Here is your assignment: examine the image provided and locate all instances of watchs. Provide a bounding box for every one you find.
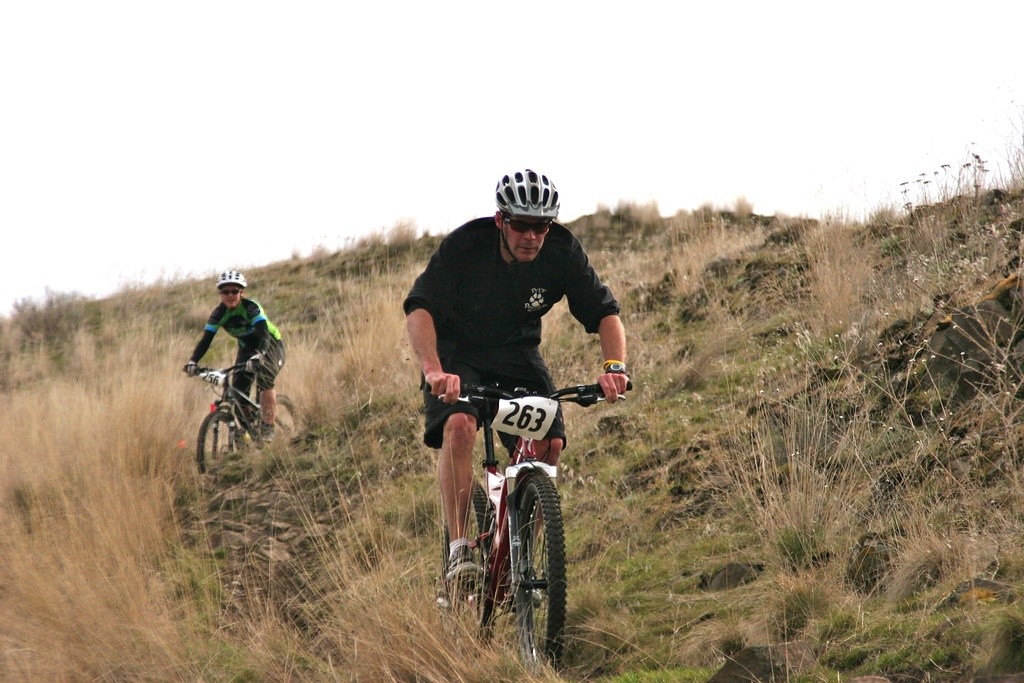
[605,364,626,374]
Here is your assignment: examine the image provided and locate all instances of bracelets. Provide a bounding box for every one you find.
[603,360,626,369]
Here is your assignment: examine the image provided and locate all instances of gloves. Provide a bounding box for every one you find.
[182,360,197,378]
[245,352,266,373]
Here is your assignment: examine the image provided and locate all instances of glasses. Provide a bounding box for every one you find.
[220,289,240,295]
[503,216,553,235]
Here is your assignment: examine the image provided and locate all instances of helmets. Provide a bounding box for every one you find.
[495,168,560,222]
[216,271,247,289]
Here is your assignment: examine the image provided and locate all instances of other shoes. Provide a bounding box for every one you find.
[259,422,275,443]
[223,445,240,457]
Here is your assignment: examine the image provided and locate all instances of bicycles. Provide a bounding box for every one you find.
[182,358,299,478]
[418,374,634,671]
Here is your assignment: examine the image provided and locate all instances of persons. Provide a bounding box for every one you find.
[402,168,632,589]
[183,270,285,436]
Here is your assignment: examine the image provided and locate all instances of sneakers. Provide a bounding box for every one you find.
[444,545,483,593]
[509,566,541,613]
